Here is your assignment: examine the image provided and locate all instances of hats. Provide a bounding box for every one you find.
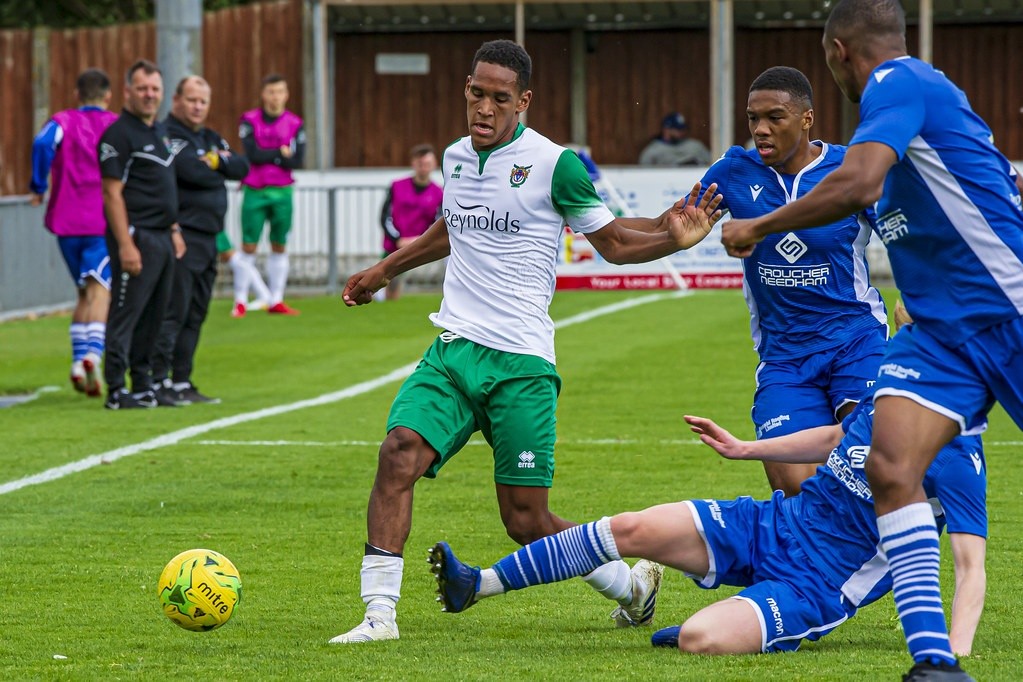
[663,113,687,129]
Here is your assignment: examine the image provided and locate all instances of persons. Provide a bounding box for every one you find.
[378,145,444,301]
[324,40,723,645]
[426,300,988,659]
[612,1,1022,681]
[29,58,306,409]
[640,112,711,170]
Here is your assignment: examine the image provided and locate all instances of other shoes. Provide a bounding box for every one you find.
[159,380,220,406]
[107,383,176,410]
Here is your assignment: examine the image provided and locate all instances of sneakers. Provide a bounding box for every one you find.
[232,302,245,317]
[610,559,663,627]
[268,302,300,315]
[652,624,682,648]
[329,608,399,644]
[902,659,976,682]
[247,300,268,310]
[427,542,482,614]
[70,353,102,397]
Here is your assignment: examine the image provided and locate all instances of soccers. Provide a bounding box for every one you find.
[157,548,242,633]
